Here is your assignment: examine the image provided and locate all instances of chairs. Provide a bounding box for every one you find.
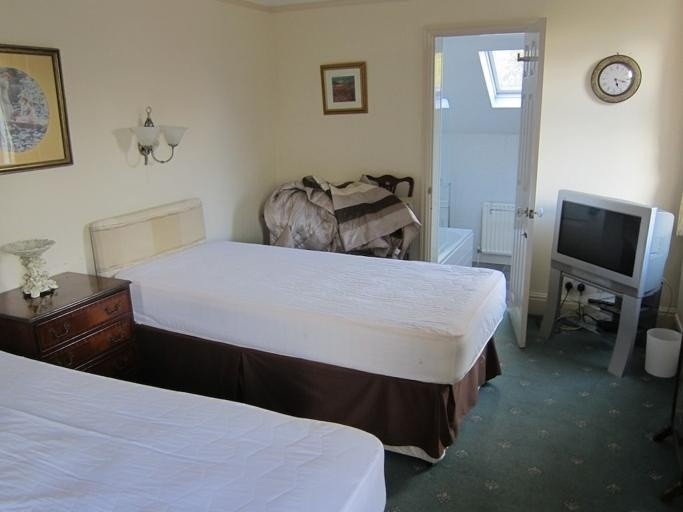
[357,174,415,260]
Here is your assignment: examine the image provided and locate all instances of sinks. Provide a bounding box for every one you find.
[438,224,472,262]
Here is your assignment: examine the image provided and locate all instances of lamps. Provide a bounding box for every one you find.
[128,105,189,167]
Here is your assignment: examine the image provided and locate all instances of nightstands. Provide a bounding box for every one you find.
[0,270,138,380]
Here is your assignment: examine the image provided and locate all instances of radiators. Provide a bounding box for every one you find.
[476,202,515,257]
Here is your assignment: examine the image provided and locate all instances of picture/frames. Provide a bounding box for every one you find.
[0,43,74,176]
[319,62,368,116]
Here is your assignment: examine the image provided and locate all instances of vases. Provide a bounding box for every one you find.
[0,237,57,298]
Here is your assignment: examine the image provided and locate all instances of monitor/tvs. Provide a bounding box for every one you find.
[550,188,674,300]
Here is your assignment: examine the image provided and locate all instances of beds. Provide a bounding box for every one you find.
[88,197,509,464]
[0,348,386,510]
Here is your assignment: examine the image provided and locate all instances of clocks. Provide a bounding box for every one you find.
[590,55,642,105]
